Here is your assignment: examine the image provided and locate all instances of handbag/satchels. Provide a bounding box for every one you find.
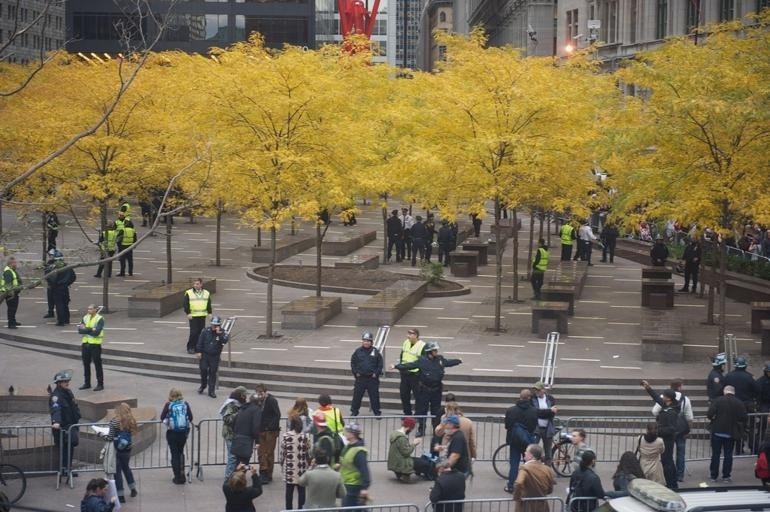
[113,431,131,451]
[511,422,532,449]
[660,413,689,441]
[230,434,254,460]
[732,420,744,439]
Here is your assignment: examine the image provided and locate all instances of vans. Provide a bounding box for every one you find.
[591,477,770,512]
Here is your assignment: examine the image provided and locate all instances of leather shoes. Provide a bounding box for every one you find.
[79,383,90,389]
[93,384,104,390]
[678,288,688,291]
[9,325,17,328]
[690,288,696,293]
[66,472,78,477]
[198,385,207,393]
[44,312,53,317]
[209,392,216,397]
[16,322,21,325]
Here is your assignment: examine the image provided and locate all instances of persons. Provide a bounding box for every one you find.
[529,238,551,302]
[47,252,77,326]
[43,248,62,318]
[382,207,459,267]
[45,371,82,478]
[160,388,194,484]
[350,328,462,436]
[77,477,116,512]
[46,211,61,253]
[221,384,477,512]
[93,203,137,278]
[97,402,137,502]
[77,303,104,392]
[184,279,229,398]
[505,353,770,511]
[0,256,22,329]
[468,209,483,237]
[559,216,770,294]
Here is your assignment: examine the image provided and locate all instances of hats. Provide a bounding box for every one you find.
[234,386,251,394]
[313,413,329,427]
[345,422,362,433]
[407,328,419,334]
[442,415,459,423]
[531,382,545,389]
[401,416,416,428]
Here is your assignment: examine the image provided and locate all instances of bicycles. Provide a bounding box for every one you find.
[491,424,589,480]
[0,461,26,512]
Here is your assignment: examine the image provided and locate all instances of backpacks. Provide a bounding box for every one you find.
[163,400,190,430]
[320,433,345,455]
[566,471,592,509]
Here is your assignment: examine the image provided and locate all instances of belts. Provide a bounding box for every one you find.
[419,385,442,392]
[538,426,546,429]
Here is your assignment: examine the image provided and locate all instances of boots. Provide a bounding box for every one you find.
[257,473,269,484]
[267,471,272,482]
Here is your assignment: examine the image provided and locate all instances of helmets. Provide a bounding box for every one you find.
[733,356,748,367]
[361,332,373,340]
[687,235,697,241]
[209,315,222,324]
[763,360,770,371]
[424,341,439,350]
[655,233,663,239]
[53,370,72,382]
[48,248,57,255]
[712,353,727,366]
[54,253,64,259]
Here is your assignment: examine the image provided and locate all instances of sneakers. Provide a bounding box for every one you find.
[118,495,126,503]
[130,489,137,497]
[710,477,717,482]
[722,477,731,482]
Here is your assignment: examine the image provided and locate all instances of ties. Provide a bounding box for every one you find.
[403,215,405,228]
